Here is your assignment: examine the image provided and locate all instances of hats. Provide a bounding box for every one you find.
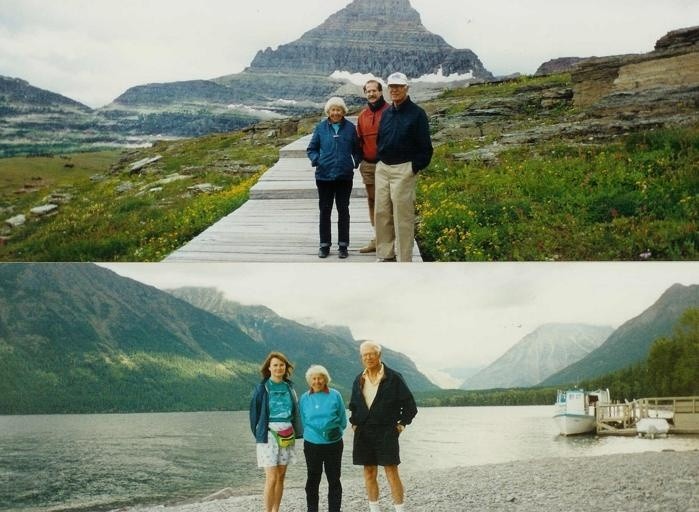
[387,72,408,85]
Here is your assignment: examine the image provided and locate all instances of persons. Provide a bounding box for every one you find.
[356,79,390,253]
[374,72,433,262]
[249,352,302,511]
[308,97,362,258]
[350,340,418,511]
[298,363,346,512]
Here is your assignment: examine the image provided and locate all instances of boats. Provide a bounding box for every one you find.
[551,389,612,436]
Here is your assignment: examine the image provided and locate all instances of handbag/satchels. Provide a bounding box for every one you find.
[268,426,297,448]
[306,424,341,441]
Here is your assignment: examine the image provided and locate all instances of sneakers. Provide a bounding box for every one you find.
[318,246,331,258]
[338,246,348,258]
[359,238,376,253]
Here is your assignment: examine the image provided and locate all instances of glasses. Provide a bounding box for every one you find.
[362,352,377,359]
